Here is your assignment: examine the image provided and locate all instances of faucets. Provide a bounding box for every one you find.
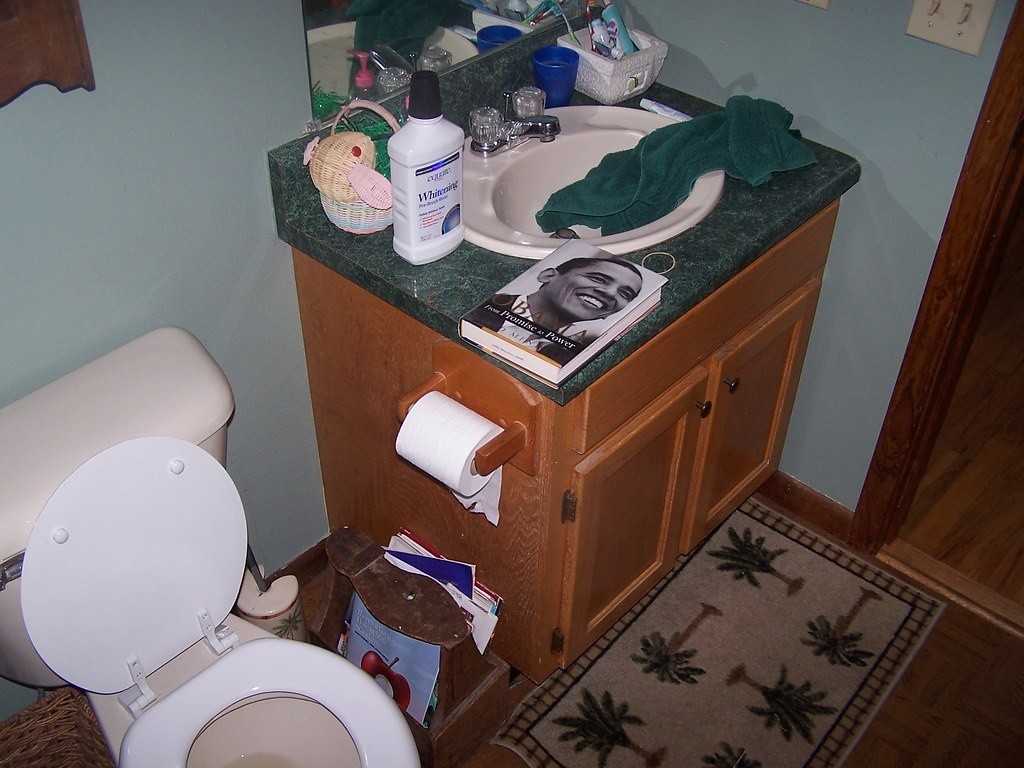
[366,42,454,96]
[465,82,563,159]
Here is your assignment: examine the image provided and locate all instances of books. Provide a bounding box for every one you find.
[457,238,670,389]
[338,527,503,727]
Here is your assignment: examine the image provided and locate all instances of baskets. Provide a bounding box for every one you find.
[320,100,401,234]
[308,132,376,203]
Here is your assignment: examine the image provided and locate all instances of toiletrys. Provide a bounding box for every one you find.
[340,48,377,103]
[383,68,470,268]
[638,97,695,122]
[446,23,478,42]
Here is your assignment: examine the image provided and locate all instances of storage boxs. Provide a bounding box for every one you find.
[309,523,510,768]
[556,25,667,105]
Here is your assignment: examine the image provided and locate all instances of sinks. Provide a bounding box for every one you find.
[306,18,480,99]
[460,101,729,263]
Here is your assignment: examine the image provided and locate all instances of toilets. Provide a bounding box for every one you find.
[0,322,427,768]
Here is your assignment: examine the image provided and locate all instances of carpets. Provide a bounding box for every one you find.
[484,493,948,768]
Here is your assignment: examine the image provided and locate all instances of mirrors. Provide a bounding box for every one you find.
[300,0,587,129]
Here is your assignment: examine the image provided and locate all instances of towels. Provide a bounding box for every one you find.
[532,94,821,239]
[0,0,98,110]
[343,0,463,96]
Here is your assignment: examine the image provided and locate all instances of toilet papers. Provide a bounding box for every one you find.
[392,389,506,528]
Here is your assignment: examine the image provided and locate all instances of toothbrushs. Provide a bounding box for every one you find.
[467,0,566,25]
[547,0,637,59]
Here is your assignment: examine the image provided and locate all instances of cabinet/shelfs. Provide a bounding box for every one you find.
[291,194,842,686]
